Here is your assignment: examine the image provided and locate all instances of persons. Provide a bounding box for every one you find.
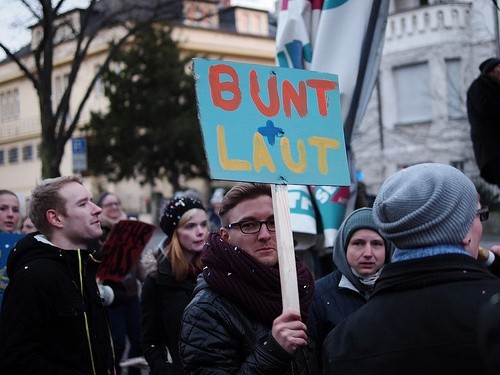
[321,163,500,375]
[0,175,117,375]
[467,58,500,188]
[20,216,38,234]
[0,190,28,307]
[311,207,394,338]
[178,182,319,375]
[98,192,141,375]
[139,195,211,375]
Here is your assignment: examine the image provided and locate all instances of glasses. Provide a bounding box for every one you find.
[476,206,490,221]
[226,217,276,234]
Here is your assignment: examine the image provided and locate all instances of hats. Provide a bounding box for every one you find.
[159,194,205,236]
[373,161,478,246]
[332,207,390,247]
